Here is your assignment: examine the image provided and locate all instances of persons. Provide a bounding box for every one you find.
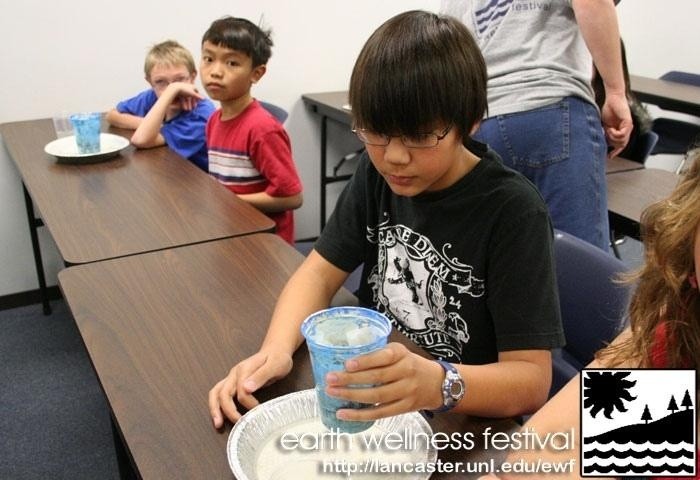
[205,10,567,429]
[591,37,653,239]
[106,40,216,173]
[448,0,634,253]
[473,143,700,480]
[198,16,303,247]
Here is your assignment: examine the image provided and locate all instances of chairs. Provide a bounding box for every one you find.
[641,129,660,165]
[257,100,288,126]
[648,71,700,171]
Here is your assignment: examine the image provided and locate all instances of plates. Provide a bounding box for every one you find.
[225,386,443,480]
[41,132,131,161]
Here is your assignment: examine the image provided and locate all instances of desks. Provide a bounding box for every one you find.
[55,231,522,477]
[300,92,646,236]
[0,113,279,316]
[628,74,700,116]
[606,166,680,262]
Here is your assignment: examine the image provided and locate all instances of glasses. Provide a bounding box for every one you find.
[352,109,456,150]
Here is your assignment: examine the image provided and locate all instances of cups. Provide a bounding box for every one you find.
[48,108,102,152]
[298,303,393,435]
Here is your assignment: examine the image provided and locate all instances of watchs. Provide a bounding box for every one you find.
[418,358,464,421]
